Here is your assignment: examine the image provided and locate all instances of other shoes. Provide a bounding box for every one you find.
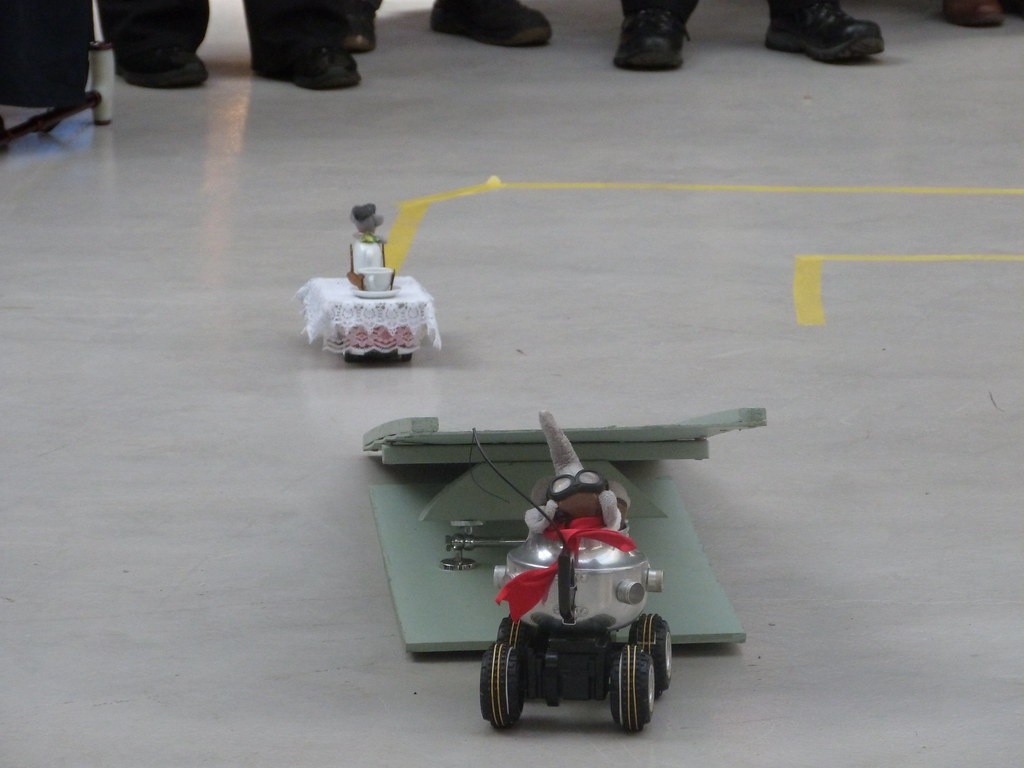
[346,12,376,53]
[111,39,208,89]
[250,51,361,90]
[944,0,1006,27]
[430,0,552,47]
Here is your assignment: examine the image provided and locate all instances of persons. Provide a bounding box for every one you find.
[942,0,1024,26]
[0,0,93,108]
[612,0,885,72]
[96,0,360,92]
[339,0,551,52]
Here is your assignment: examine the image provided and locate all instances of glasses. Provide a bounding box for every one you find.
[546,469,609,501]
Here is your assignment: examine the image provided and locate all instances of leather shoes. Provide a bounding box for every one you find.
[613,0,699,71]
[765,0,884,62]
